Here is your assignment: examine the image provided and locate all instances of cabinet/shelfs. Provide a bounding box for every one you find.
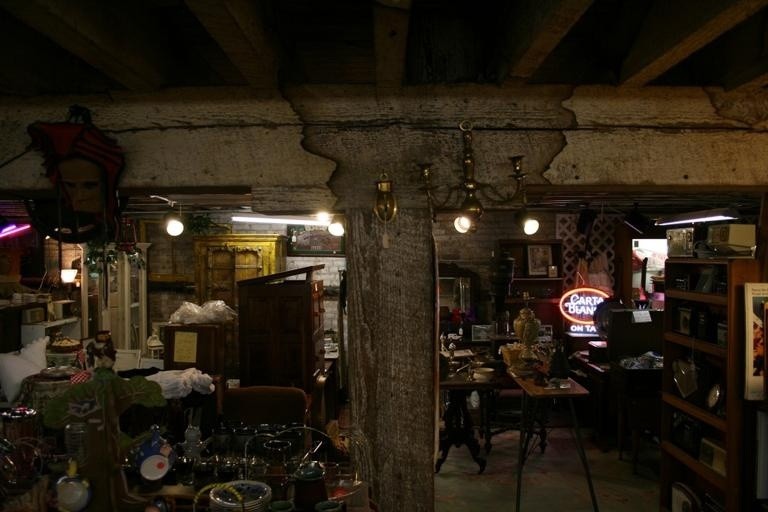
[658,257,764,511]
[497,238,564,336]
[20,233,326,424]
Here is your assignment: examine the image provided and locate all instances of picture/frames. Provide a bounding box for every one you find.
[286,224,346,257]
[743,281,768,402]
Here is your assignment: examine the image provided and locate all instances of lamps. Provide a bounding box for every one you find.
[443,209,477,235]
[328,216,346,236]
[163,212,187,237]
[514,210,540,236]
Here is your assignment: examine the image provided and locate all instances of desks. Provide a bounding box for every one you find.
[434,370,494,473]
[505,366,600,512]
